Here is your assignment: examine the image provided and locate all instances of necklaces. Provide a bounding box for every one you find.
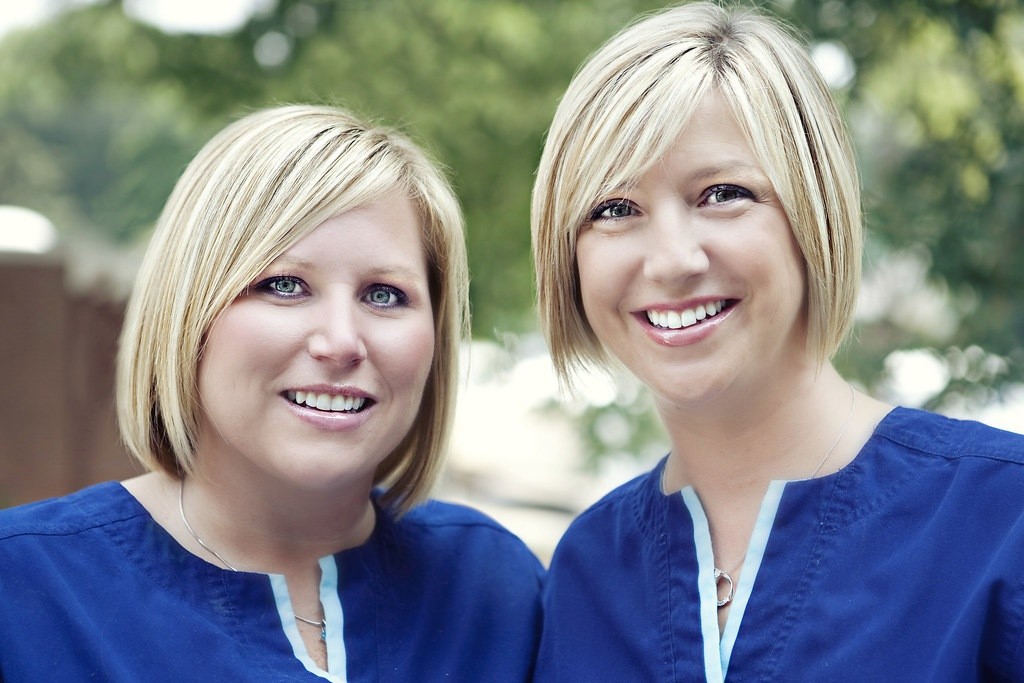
[662,389,856,608]
[178,476,327,643]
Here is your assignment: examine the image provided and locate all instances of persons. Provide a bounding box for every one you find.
[0,104,543,683]
[530,0,1023,683]
